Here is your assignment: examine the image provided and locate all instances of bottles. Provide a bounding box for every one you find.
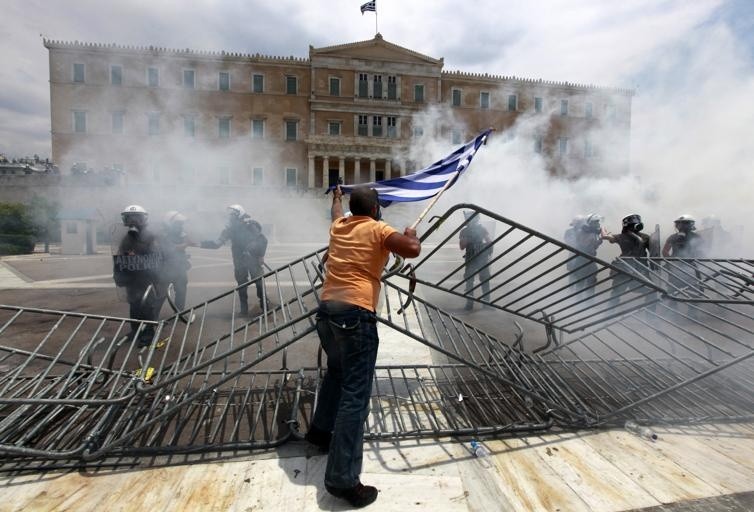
[624,420,658,441]
[470,441,494,468]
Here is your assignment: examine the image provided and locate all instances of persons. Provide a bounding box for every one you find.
[563,212,730,332]
[156,212,191,314]
[302,182,422,508]
[23,164,33,174]
[458,210,498,311]
[111,204,162,348]
[191,203,269,319]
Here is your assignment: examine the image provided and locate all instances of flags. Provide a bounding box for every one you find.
[360,0,376,16]
[322,131,491,210]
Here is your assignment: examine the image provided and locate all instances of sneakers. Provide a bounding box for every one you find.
[325,482,379,509]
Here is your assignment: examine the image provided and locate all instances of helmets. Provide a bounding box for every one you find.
[120,204,149,216]
[163,210,186,224]
[227,204,247,219]
[569,212,721,228]
